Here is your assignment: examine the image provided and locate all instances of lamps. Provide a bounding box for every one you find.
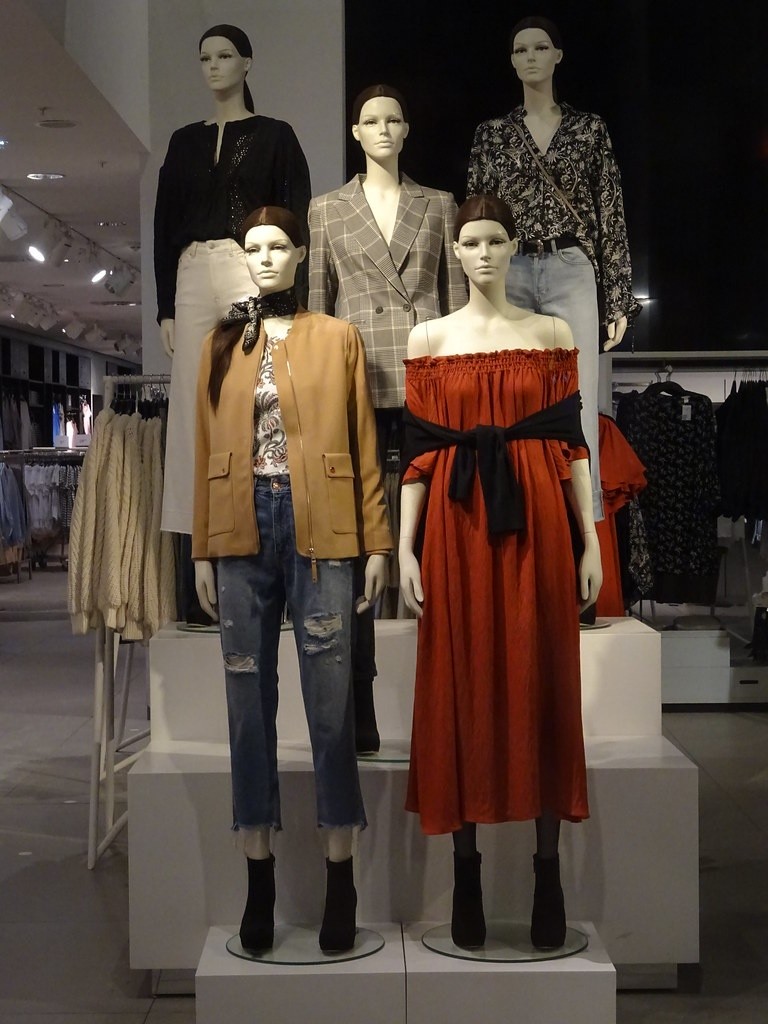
[1,288,143,359]
[0,183,142,296]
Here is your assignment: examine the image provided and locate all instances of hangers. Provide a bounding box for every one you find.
[24,454,85,468]
[109,372,168,420]
[649,366,685,397]
[731,368,768,392]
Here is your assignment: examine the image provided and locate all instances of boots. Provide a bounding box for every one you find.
[239,855,276,956]
[449,849,489,953]
[318,855,358,954]
[528,853,567,951]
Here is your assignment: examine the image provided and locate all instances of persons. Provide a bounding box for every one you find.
[467,16,632,623]
[308,85,459,621]
[191,206,392,959]
[398,193,603,956]
[154,24,312,627]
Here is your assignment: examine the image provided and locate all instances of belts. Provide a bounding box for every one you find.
[517,233,582,257]
